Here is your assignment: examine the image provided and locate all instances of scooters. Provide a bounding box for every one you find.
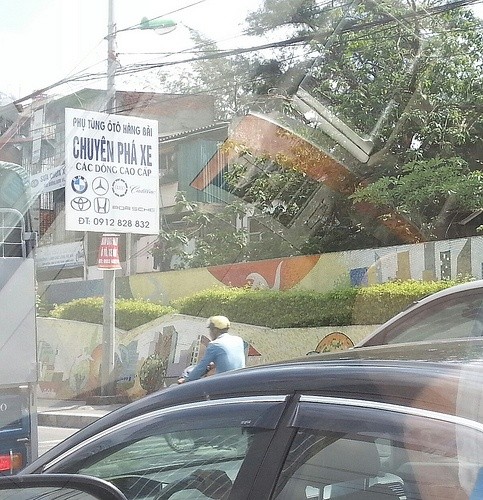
[162,364,214,453]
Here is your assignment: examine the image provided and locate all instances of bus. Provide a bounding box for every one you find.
[0,160,42,477]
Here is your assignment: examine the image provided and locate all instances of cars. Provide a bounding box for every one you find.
[349,278,483,349]
[18,337,481,499]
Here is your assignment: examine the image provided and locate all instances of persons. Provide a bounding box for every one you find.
[178,315,246,386]
[190,425,315,497]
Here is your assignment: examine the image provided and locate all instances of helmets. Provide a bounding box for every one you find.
[207,315,231,329]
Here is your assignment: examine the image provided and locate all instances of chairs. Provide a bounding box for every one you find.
[316,430,401,500]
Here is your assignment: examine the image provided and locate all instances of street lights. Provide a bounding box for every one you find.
[101,0,178,396]
